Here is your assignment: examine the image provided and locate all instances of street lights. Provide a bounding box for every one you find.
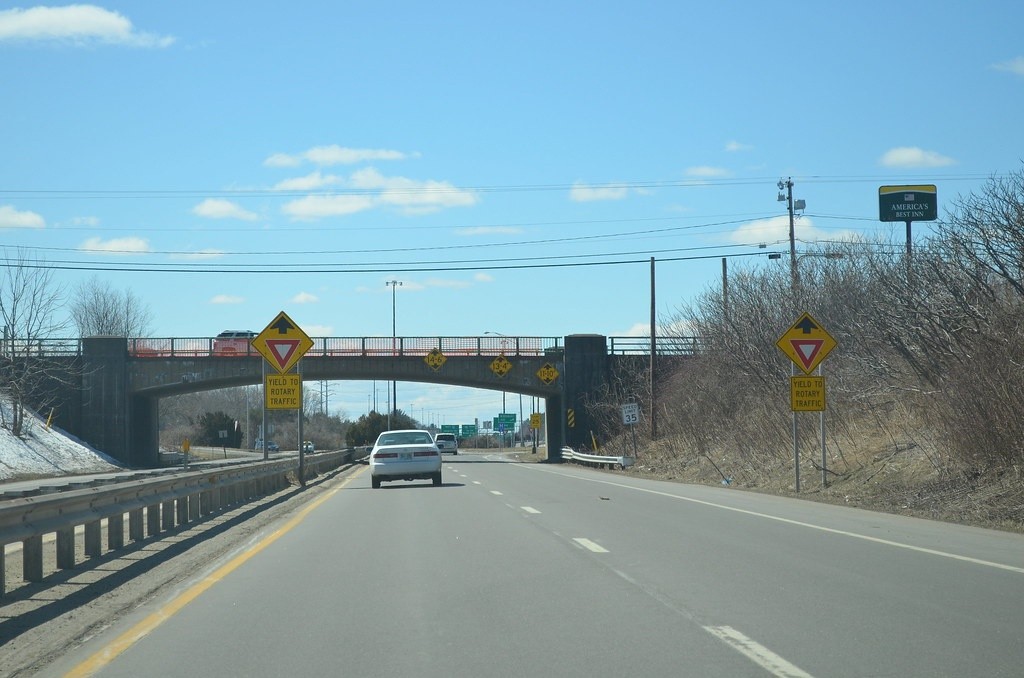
[484,332,524,447]
[386,280,402,416]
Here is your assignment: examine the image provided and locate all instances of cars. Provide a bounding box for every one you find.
[267,441,280,452]
[435,433,458,455]
[366,429,444,489]
[214,330,259,338]
[303,441,315,453]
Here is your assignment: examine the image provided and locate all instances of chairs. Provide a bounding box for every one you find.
[385,439,396,445]
[415,438,426,444]
